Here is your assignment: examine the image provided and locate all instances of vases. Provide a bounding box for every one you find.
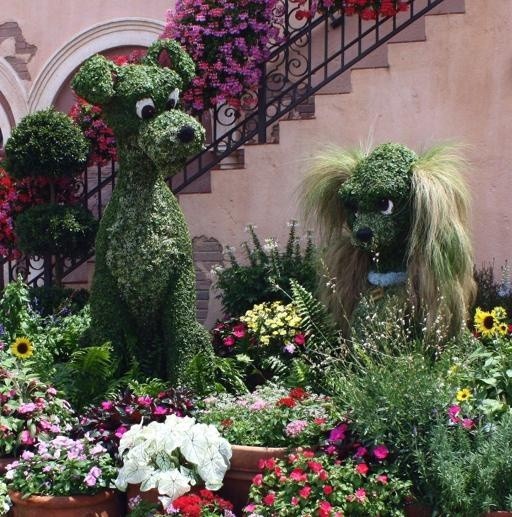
[128,482,166,513]
[213,437,325,517]
[8,488,118,517]
[0,458,20,476]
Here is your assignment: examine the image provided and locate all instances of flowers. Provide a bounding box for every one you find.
[0,336,81,458]
[5,431,118,496]
[127,490,236,517]
[242,444,413,517]
[81,377,198,438]
[115,414,233,502]
[199,380,354,446]
[209,300,304,357]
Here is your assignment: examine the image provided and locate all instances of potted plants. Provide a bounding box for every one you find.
[269,258,511,517]
